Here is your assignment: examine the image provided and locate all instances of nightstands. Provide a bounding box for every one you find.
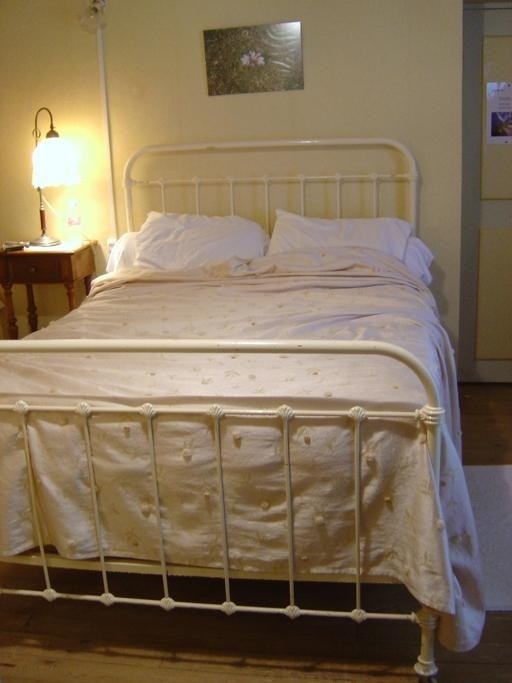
[0,241,95,340]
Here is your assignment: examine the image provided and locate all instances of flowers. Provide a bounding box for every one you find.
[240,49,265,69]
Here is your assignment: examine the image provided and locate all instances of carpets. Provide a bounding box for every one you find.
[460,463,511,614]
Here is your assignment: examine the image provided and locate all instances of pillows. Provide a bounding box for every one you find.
[107,231,142,277]
[266,207,416,263]
[134,209,267,281]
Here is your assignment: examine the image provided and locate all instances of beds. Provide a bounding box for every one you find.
[0,136,469,676]
